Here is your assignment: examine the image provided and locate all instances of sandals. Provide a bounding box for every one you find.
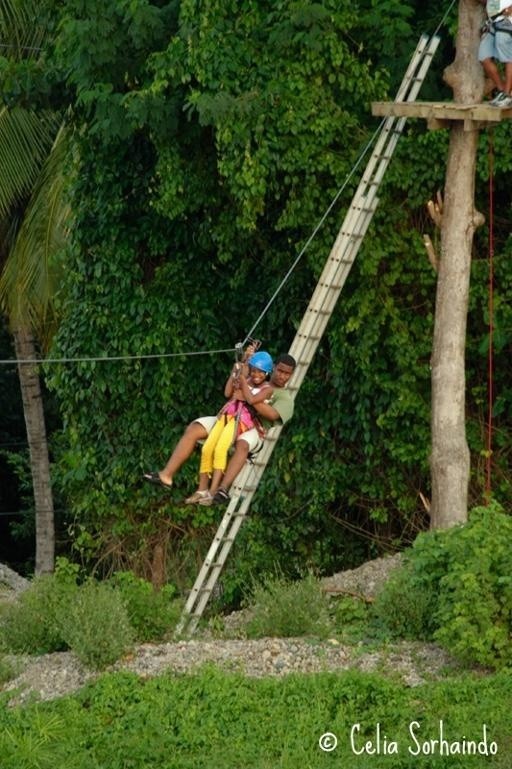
[185,489,229,506]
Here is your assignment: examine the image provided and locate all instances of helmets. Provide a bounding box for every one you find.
[247,351,273,372]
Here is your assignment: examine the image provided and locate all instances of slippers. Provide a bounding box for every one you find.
[142,472,171,489]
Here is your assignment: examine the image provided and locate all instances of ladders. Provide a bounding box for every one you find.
[169,35,446,650]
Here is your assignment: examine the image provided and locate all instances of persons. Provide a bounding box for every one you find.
[184,351,275,506]
[477,0,512,107]
[141,343,297,505]
